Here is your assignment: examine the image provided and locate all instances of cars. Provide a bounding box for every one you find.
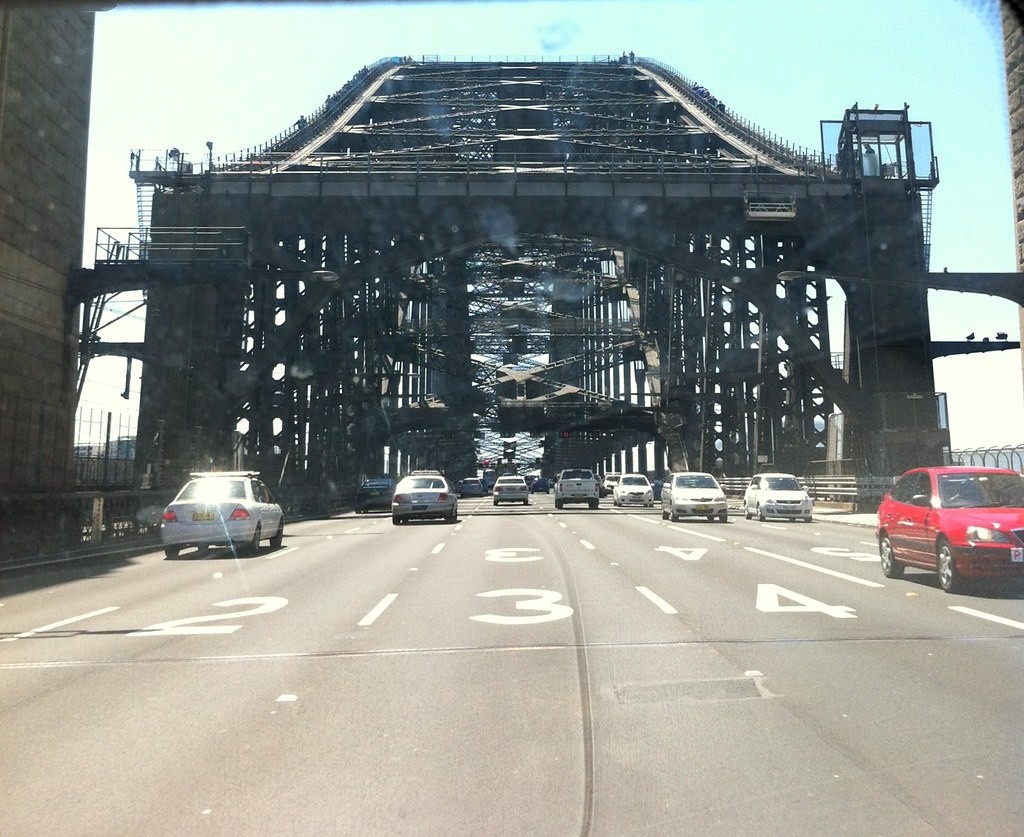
[160,477,285,558]
[611,474,654,507]
[492,476,528,505]
[743,473,812,523]
[452,480,463,493]
[661,472,728,522]
[603,475,620,494]
[459,478,490,498]
[593,474,605,498]
[876,465,1024,594]
[392,476,460,524]
[354,479,396,514]
[531,479,550,494]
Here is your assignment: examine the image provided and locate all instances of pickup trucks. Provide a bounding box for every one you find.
[554,469,600,510]
